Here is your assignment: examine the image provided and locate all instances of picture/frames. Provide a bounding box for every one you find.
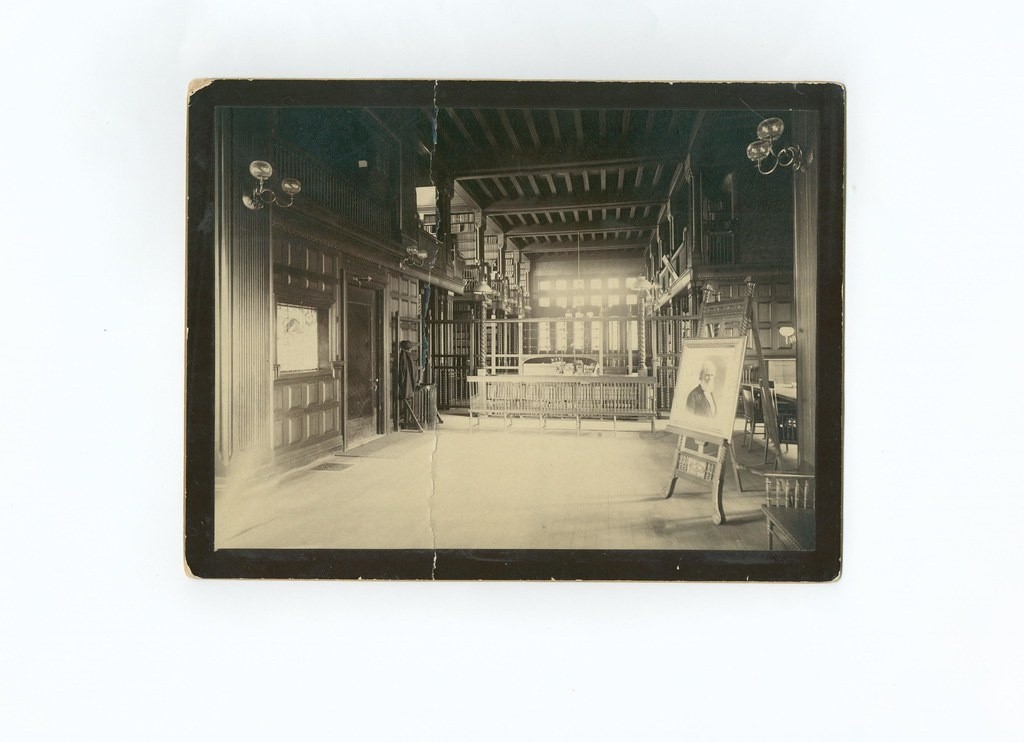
[664,333,749,443]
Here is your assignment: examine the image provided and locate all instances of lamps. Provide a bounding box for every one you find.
[400,246,428,271]
[744,114,817,176]
[239,158,302,213]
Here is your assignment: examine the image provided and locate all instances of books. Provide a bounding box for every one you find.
[420,207,528,312]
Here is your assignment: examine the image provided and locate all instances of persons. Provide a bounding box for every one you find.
[685,360,719,420]
